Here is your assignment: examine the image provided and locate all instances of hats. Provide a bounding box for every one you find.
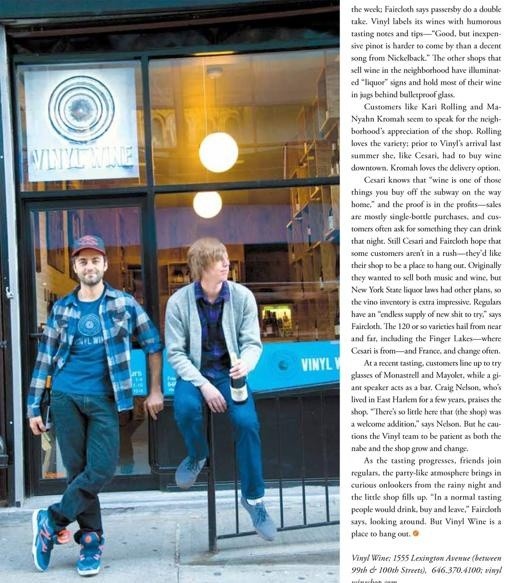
[71,234,106,255]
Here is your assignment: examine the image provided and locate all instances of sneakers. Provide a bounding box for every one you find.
[240,497,276,541]
[32,508,71,571]
[76,531,105,576]
[175,455,207,490]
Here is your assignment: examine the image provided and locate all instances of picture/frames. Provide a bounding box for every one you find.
[33,181,81,284]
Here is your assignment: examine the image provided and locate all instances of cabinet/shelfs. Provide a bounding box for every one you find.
[161,258,241,295]
[282,63,340,341]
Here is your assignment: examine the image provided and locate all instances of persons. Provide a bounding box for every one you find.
[25,234,166,576]
[164,237,277,541]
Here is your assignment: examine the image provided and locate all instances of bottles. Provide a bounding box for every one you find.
[263,308,290,339]
[228,351,250,406]
[329,137,340,176]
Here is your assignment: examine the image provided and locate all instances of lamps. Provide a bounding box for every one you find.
[199,65,239,173]
[192,191,223,219]
